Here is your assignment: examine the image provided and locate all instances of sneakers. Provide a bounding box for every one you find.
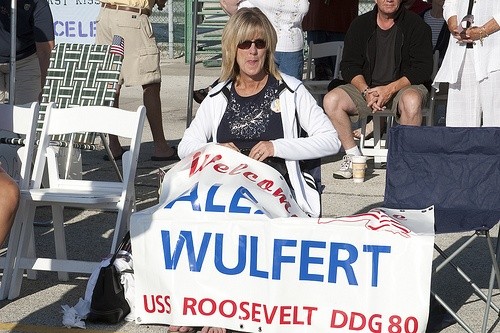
[333,154,368,179]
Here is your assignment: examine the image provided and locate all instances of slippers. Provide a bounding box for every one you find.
[152,146,180,160]
[104,148,128,161]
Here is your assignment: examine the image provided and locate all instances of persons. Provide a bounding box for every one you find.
[192,0,311,104]
[422,0,451,65]
[353,0,428,140]
[177,5,341,216]
[96,0,180,161]
[0,0,56,104]
[0,161,21,248]
[431,0,500,127]
[323,0,432,179]
[302,0,358,81]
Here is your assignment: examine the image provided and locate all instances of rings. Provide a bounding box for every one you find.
[257,152,262,156]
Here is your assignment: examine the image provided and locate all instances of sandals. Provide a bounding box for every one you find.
[193,88,208,103]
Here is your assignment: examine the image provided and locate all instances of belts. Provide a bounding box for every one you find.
[101,3,150,16]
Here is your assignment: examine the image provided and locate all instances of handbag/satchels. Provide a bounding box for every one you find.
[88,231,131,325]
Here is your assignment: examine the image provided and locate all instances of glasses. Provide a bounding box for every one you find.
[238,38,265,50]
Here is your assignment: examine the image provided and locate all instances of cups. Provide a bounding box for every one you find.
[352,156,367,183]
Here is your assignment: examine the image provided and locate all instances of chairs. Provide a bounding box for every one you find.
[381,123,500,333]
[34,35,125,225]
[0,102,40,300]
[359,51,440,164]
[7,101,146,302]
[301,40,344,95]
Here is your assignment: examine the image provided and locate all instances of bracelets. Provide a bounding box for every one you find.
[480,27,487,39]
[362,87,369,95]
